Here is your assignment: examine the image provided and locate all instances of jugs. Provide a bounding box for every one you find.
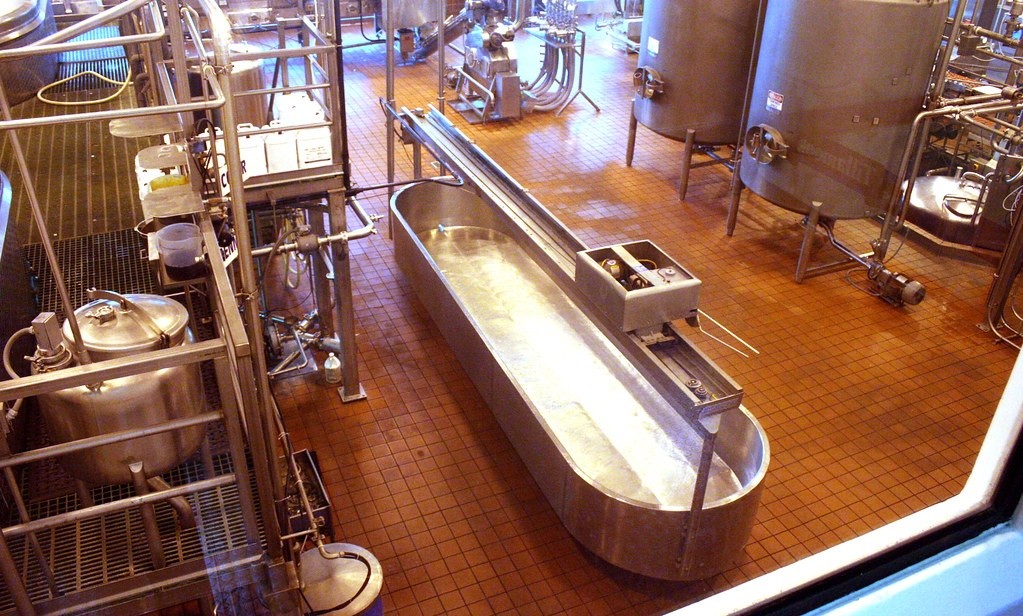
[157,223,205,281]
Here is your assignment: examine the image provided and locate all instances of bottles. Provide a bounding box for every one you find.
[324,352,342,383]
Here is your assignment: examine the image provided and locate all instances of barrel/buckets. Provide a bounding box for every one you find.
[164,89,334,197]
[188,43,269,128]
